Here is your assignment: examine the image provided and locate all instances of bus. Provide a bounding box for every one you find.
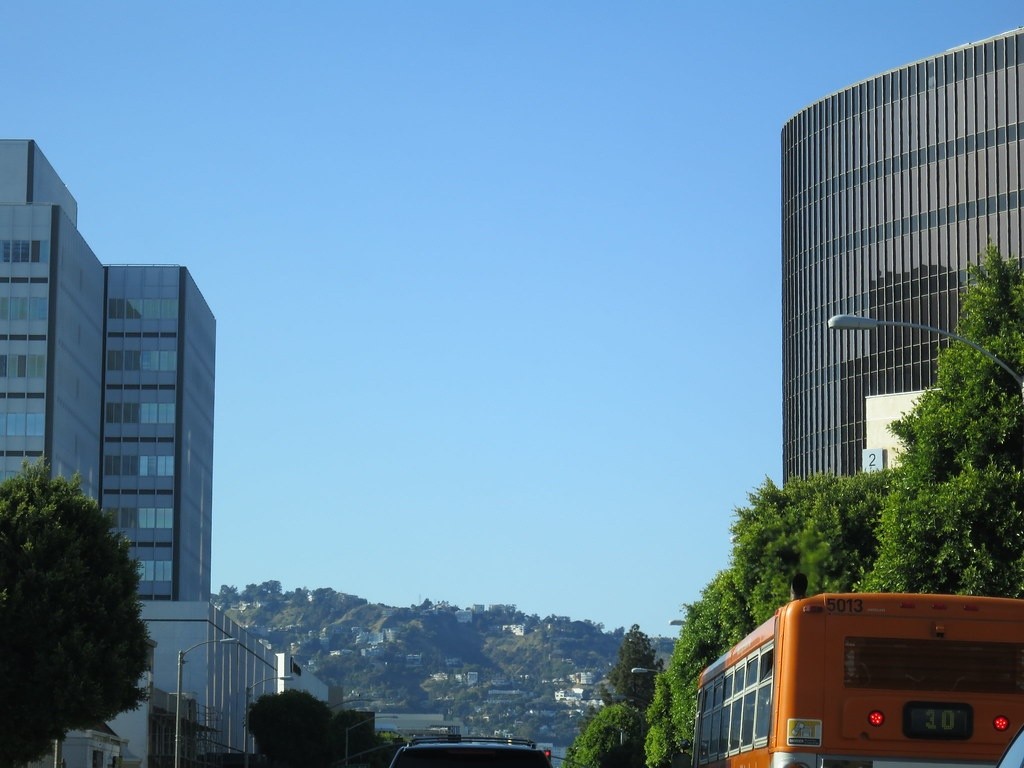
[670,592,1024,768]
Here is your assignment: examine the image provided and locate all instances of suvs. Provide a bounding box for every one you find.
[389,735,553,768]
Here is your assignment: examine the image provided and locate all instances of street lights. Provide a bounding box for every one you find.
[581,697,644,739]
[175,638,239,768]
[244,675,293,768]
[570,713,624,747]
[344,714,398,768]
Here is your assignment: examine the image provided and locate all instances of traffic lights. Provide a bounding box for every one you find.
[543,750,550,763]
